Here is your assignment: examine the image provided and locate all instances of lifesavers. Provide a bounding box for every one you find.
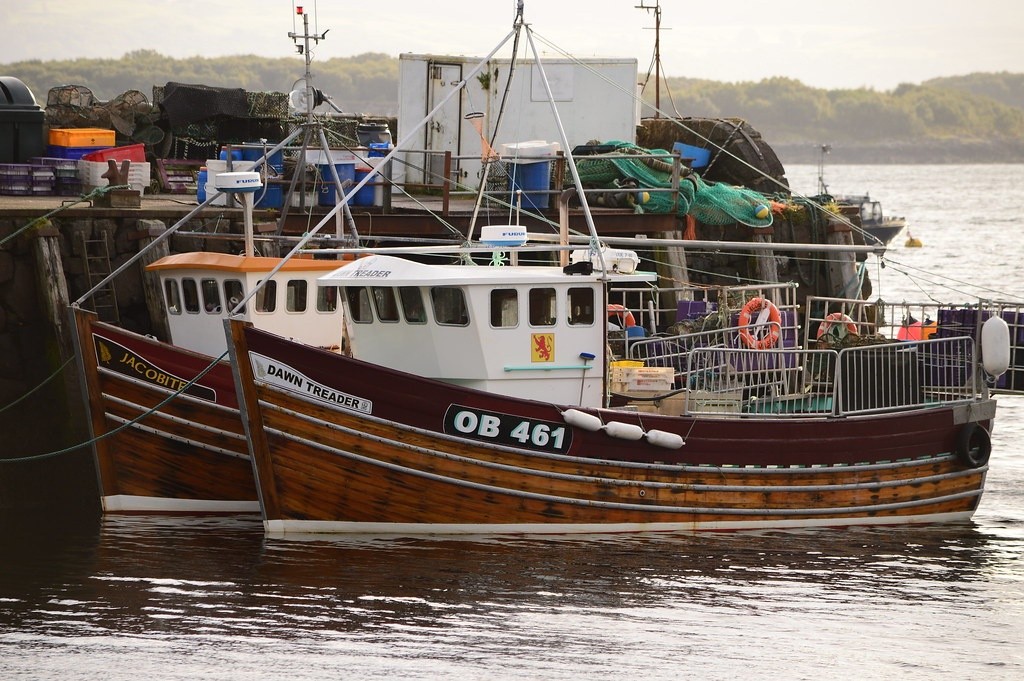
[609,304,634,328]
[818,311,858,349]
[957,423,991,468]
[738,298,783,350]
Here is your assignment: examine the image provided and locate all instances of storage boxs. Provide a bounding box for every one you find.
[607,359,675,391]
[0,128,150,198]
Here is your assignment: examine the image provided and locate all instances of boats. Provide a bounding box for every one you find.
[223,0,1010,538]
[63,0,803,515]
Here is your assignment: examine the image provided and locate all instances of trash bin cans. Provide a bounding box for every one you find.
[508,161,550,208]
[356,169,377,206]
[315,163,356,206]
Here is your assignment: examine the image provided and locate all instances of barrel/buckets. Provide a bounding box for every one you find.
[316,164,354,205]
[198,167,207,203]
[243,143,282,208]
[355,168,375,205]
[508,162,550,208]
[220,146,243,160]
[362,158,384,206]
